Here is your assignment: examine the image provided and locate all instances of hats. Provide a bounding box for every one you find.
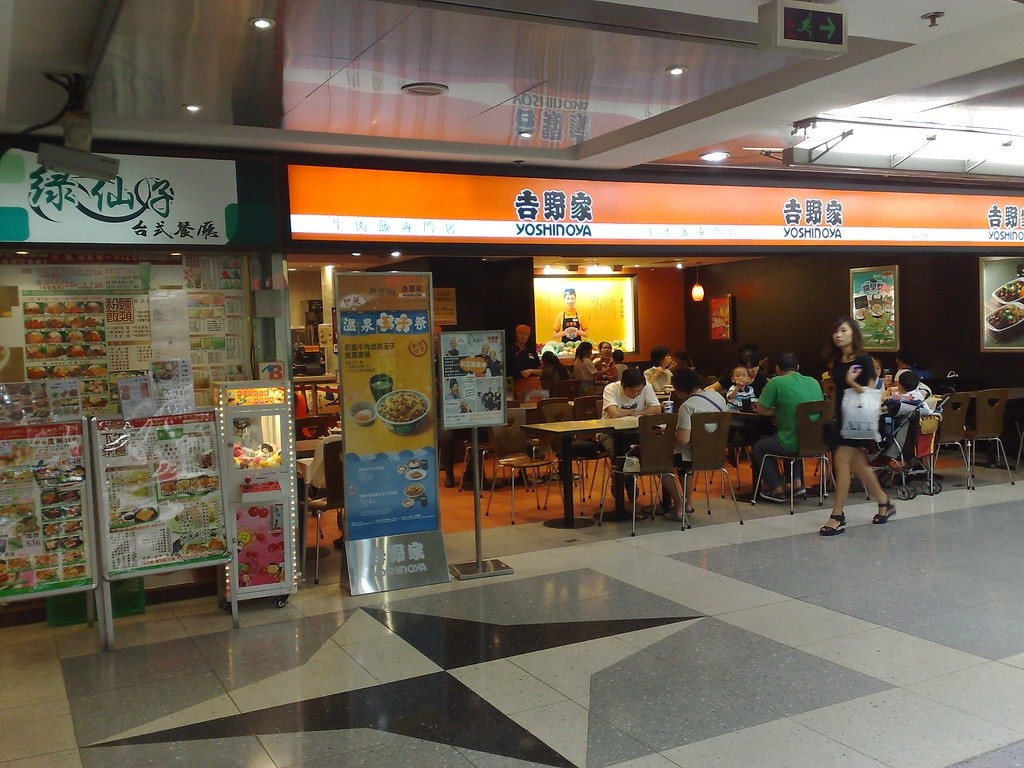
[516,325,530,333]
[564,288,576,296]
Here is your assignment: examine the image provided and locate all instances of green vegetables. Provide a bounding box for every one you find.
[1002,309,1013,321]
[1004,282,1018,294]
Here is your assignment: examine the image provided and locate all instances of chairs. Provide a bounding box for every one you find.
[294,413,344,584]
[439,363,1024,537]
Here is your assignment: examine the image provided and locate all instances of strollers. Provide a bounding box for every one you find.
[865,385,956,501]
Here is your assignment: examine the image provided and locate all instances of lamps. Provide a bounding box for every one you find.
[692,262,704,302]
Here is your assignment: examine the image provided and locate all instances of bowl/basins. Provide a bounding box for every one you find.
[404,484,425,500]
[397,465,407,474]
[375,390,430,434]
[349,401,377,427]
[407,460,419,469]
[402,499,414,508]
[565,328,577,339]
[330,431,342,435]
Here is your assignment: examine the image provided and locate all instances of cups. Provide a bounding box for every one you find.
[421,460,428,469]
[663,401,674,414]
[883,391,890,401]
[664,385,673,393]
[891,387,898,396]
[885,375,892,387]
[370,373,393,402]
[337,421,341,428]
[420,496,427,506]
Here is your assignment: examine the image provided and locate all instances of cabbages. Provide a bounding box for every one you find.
[541,341,556,354]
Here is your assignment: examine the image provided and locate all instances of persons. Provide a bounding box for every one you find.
[506,325,544,404]
[657,367,726,521]
[704,342,935,502]
[642,347,694,392]
[553,289,587,344]
[596,369,663,502]
[574,342,628,386]
[539,351,569,382]
[447,338,503,413]
[820,315,898,535]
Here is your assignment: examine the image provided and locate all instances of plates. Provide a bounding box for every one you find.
[985,302,1024,334]
[406,469,427,481]
[992,277,1024,304]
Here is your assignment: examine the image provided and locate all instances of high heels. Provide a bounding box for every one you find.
[819,510,846,536]
[873,497,897,524]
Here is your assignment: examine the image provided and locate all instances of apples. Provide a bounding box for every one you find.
[556,341,626,354]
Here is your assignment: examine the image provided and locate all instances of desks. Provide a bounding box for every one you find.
[728,398,776,502]
[654,388,677,399]
[880,389,942,409]
[504,400,583,480]
[520,413,673,531]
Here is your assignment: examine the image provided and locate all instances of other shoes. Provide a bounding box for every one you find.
[789,486,807,498]
[624,476,639,501]
[664,512,688,522]
[686,506,695,513]
[444,472,455,488]
[760,491,787,502]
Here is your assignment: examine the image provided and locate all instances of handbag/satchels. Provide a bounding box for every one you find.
[841,386,882,443]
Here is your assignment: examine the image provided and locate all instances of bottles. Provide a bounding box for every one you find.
[609,362,613,367]
[743,394,751,411]
[601,363,605,370]
